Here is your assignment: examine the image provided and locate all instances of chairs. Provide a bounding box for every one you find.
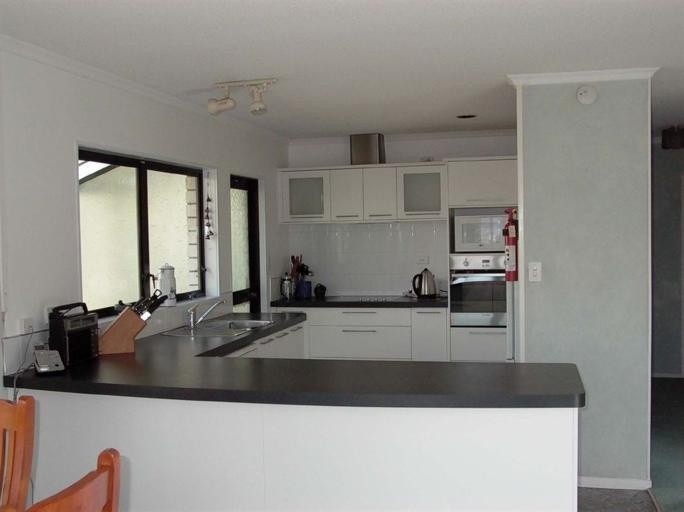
[28,446,124,512]
[0,393,44,511]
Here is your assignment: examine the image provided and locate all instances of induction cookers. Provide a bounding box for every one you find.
[328,294,412,303]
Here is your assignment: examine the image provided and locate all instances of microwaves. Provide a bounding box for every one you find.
[454,215,516,253]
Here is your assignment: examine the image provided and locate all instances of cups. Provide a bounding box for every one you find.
[296,281,312,299]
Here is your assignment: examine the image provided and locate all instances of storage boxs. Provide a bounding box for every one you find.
[350,132,386,165]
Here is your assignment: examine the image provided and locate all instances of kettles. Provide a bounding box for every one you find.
[413,268,437,300]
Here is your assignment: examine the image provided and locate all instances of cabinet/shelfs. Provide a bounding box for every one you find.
[222,320,308,358]
[449,325,519,361]
[445,156,519,209]
[362,162,399,225]
[329,165,363,225]
[398,160,450,224]
[276,307,413,362]
[276,165,331,225]
[410,306,448,362]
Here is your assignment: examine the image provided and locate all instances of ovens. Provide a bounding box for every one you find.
[449,253,506,326]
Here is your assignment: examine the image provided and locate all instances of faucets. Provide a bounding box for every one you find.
[188,299,225,329]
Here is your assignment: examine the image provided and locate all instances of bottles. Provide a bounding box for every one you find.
[281,272,292,304]
[158,263,176,307]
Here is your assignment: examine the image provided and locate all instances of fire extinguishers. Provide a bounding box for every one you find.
[503,208,518,281]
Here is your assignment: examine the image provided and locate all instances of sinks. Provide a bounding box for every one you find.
[162,329,251,337]
[198,319,275,330]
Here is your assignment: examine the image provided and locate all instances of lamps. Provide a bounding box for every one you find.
[207,78,278,115]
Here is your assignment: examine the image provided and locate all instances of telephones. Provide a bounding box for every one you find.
[33,350,65,373]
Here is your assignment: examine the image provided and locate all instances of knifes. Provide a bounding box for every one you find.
[131,289,168,321]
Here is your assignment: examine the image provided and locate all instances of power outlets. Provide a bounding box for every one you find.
[24,318,35,333]
[415,255,429,265]
